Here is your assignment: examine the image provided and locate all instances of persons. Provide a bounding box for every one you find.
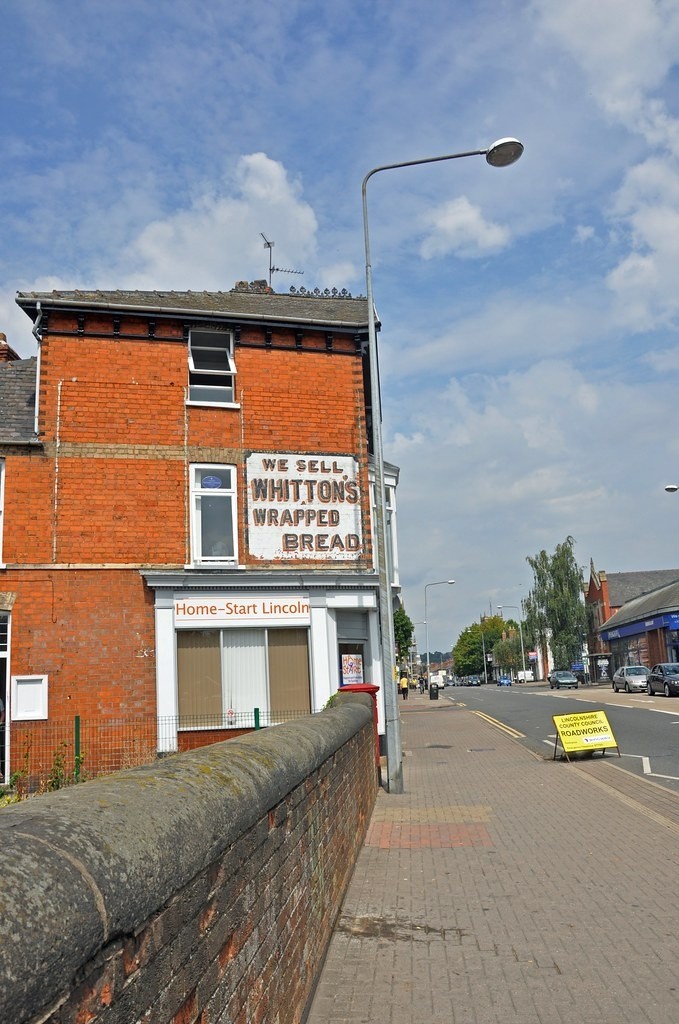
[400,674,409,700]
[348,657,353,673]
[412,674,428,694]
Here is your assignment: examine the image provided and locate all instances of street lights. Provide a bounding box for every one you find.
[496,605,527,684]
[424,580,456,695]
[358,137,526,801]
[467,630,488,685]
[411,622,426,682]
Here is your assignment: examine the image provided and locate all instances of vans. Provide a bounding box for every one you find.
[430,676,445,689]
[514,671,534,683]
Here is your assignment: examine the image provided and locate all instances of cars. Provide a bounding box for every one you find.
[550,671,578,690]
[496,676,512,687]
[442,674,481,687]
[613,666,651,693]
[647,663,679,697]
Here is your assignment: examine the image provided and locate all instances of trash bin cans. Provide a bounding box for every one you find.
[585,673,590,682]
[430,683,438,700]
